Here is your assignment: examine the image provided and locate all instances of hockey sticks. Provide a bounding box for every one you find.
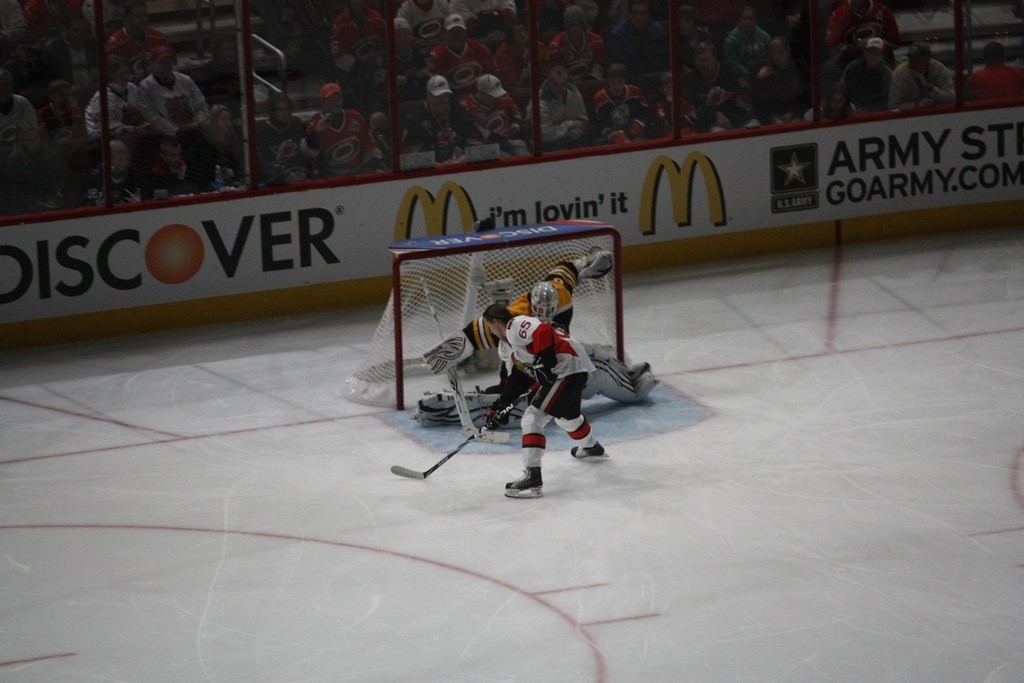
[390,379,540,480]
[419,277,512,445]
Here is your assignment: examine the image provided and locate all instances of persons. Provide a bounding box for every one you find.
[414,245,656,431]
[483,303,610,498]
[0,0,1024,217]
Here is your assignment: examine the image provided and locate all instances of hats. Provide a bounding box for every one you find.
[903,41,932,61]
[562,5,587,33]
[477,75,507,98]
[444,14,467,31]
[864,36,885,54]
[147,43,180,66]
[319,83,342,103]
[395,18,411,33]
[426,76,453,96]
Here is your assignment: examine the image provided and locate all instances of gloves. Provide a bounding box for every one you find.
[486,407,509,429]
[533,359,556,385]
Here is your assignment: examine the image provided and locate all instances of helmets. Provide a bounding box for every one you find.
[531,282,558,309]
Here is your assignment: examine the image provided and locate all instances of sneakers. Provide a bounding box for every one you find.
[505,468,543,498]
[571,441,610,461]
